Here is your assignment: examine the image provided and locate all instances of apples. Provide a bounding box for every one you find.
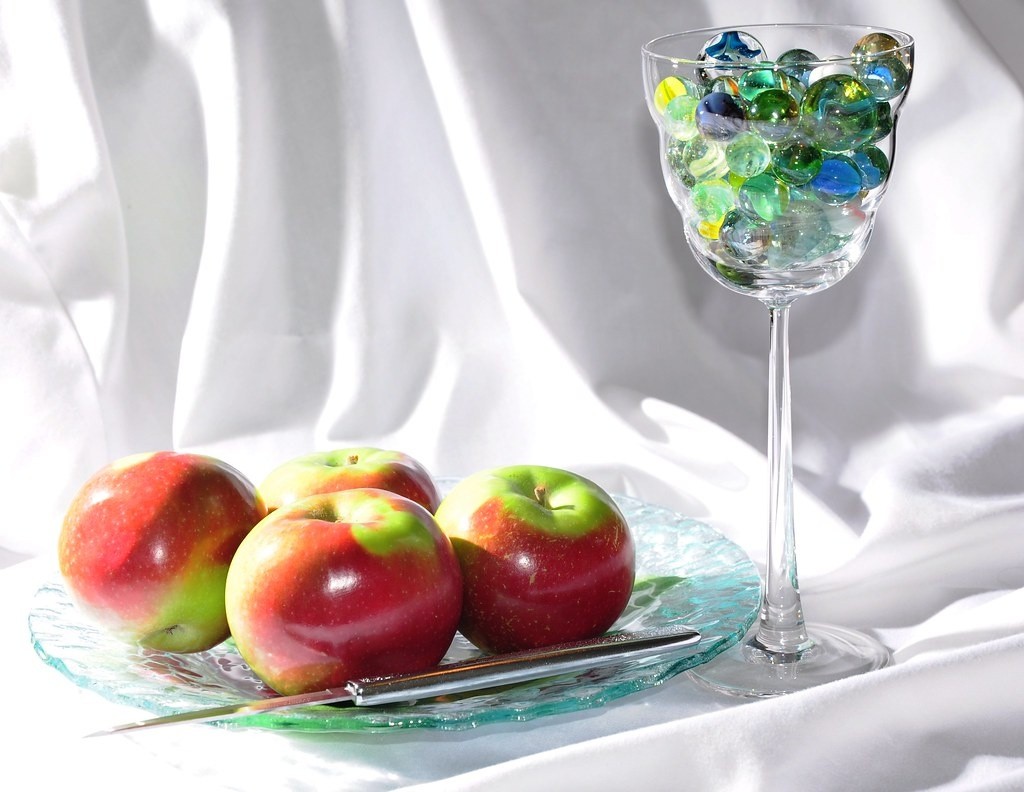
[257,447,442,516]
[432,463,635,656]
[55,452,268,653]
[225,488,462,695]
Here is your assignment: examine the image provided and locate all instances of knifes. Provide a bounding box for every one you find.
[84,624,700,738]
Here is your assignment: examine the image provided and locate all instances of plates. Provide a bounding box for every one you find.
[28,477,763,732]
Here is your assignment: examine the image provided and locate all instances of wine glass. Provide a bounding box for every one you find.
[641,23,915,700]
